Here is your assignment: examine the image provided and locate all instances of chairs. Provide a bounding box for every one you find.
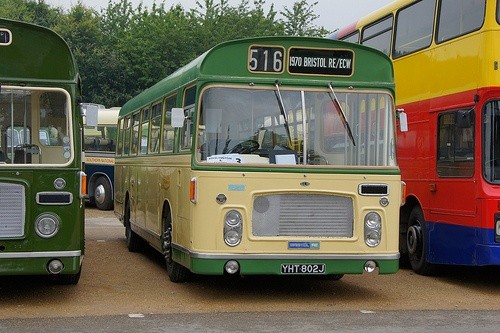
[210,138,241,156]
[14,150,31,164]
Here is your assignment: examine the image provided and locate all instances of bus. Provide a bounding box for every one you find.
[0,17,87,287]
[325,0,500,276]
[113,35,408,284]
[81,103,205,211]
